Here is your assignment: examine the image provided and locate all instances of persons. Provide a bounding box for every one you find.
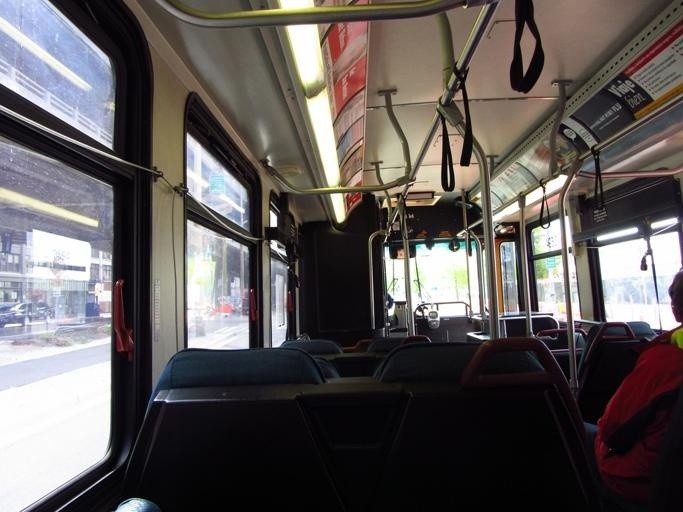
[393,246,404,258]
[386,294,393,310]
[592,271,683,504]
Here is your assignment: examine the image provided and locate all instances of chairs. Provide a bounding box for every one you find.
[499,317,557,339]
[361,335,432,355]
[280,338,343,355]
[599,380,682,512]
[371,341,546,384]
[123,348,326,418]
[576,324,657,385]
